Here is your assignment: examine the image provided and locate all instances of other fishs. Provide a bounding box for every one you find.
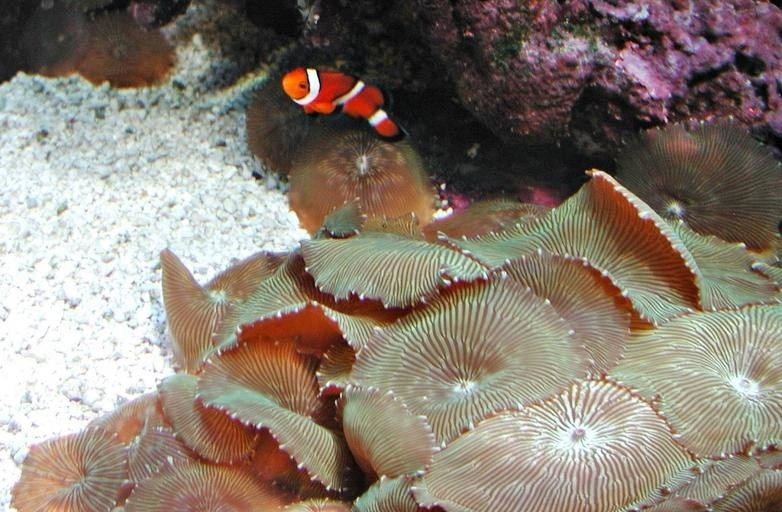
[281,66,408,145]
